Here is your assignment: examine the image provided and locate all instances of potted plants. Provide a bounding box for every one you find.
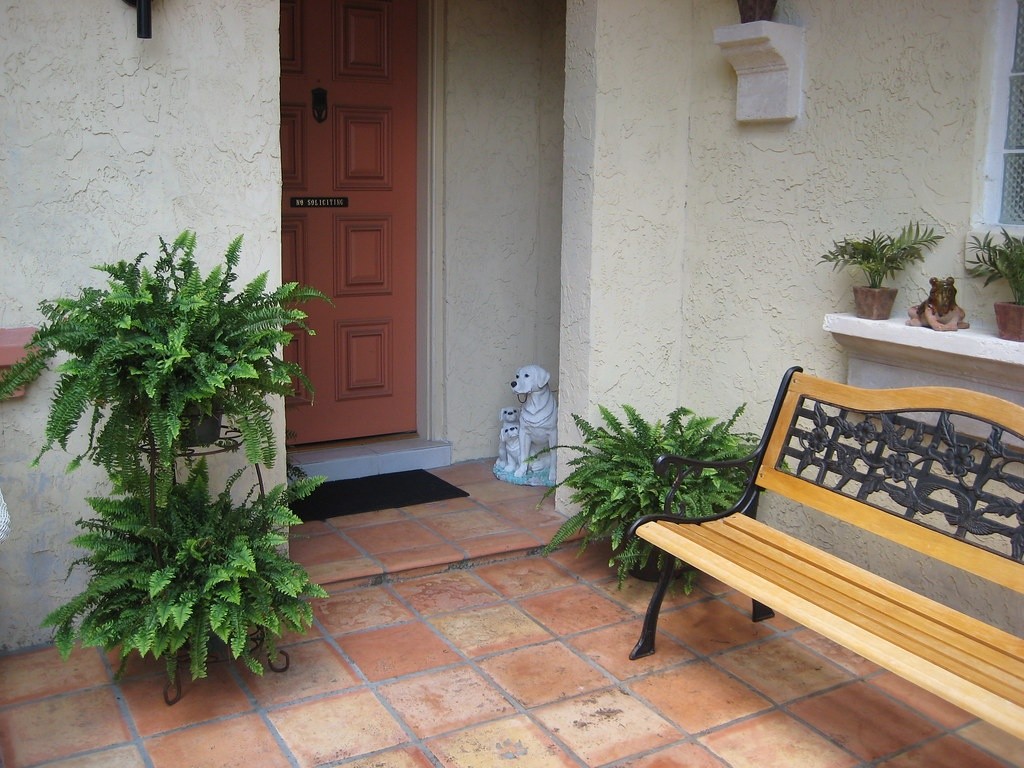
[814,220,946,320]
[954,225,1024,345]
[34,456,331,692]
[523,401,789,597]
[0,228,337,510]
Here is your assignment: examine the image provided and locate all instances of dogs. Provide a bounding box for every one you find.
[496,407,520,468]
[497,425,520,471]
[509,363,556,482]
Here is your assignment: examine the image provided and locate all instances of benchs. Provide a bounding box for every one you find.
[630,364,1024,746]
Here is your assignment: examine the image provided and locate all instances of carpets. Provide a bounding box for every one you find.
[290,468,470,524]
